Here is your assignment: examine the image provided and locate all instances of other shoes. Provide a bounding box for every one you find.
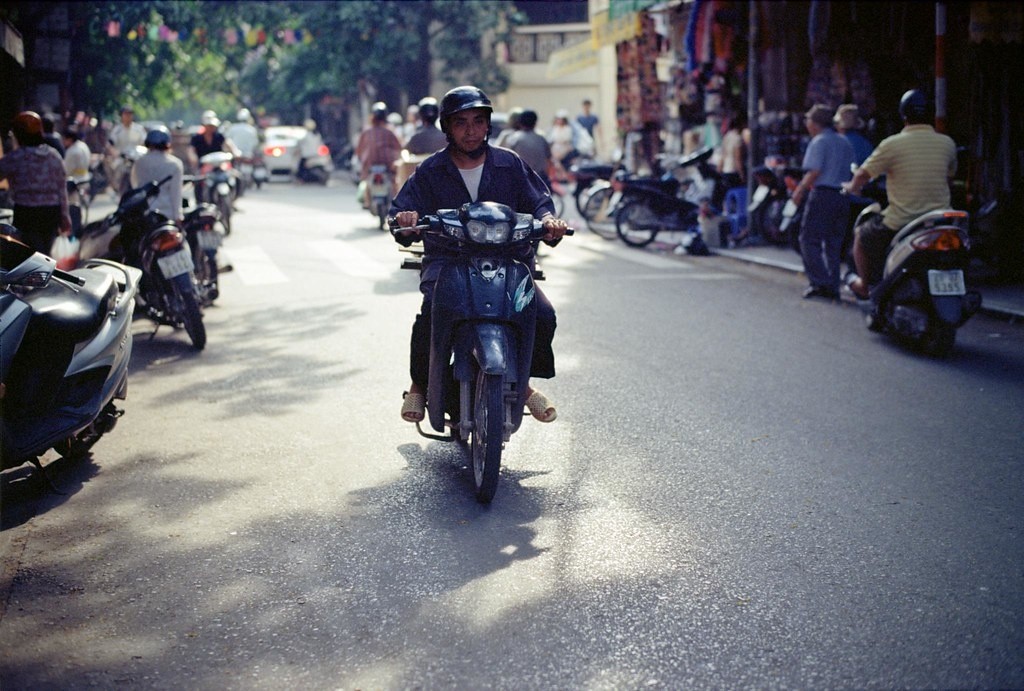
[801,283,823,299]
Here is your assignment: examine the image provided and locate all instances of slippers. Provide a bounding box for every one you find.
[524,388,557,422]
[401,394,426,423]
[845,273,870,301]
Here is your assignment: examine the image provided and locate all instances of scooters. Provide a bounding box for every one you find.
[361,164,394,230]
[841,162,981,352]
[300,154,334,186]
[91,139,254,352]
[0,177,143,495]
[605,146,728,248]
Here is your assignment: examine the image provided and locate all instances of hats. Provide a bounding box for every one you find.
[201,110,220,127]
[805,103,835,123]
[834,104,861,128]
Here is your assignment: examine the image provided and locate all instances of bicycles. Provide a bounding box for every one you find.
[551,153,667,242]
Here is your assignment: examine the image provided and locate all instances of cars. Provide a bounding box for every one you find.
[259,125,307,173]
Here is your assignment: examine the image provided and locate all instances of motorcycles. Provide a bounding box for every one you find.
[389,202,574,503]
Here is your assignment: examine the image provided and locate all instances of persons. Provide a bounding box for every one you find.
[387,85,568,424]
[292,118,323,185]
[841,90,960,300]
[577,100,600,138]
[185,108,257,205]
[548,111,573,160]
[107,106,148,166]
[111,130,184,272]
[792,103,855,301]
[493,109,555,197]
[833,105,873,284]
[354,97,450,210]
[0,110,109,257]
[675,117,748,256]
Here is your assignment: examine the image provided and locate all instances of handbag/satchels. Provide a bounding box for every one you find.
[50,233,81,271]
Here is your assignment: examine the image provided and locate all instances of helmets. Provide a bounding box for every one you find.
[17,111,43,138]
[419,97,440,119]
[439,86,493,132]
[146,126,171,143]
[369,102,388,118]
[900,87,935,116]
